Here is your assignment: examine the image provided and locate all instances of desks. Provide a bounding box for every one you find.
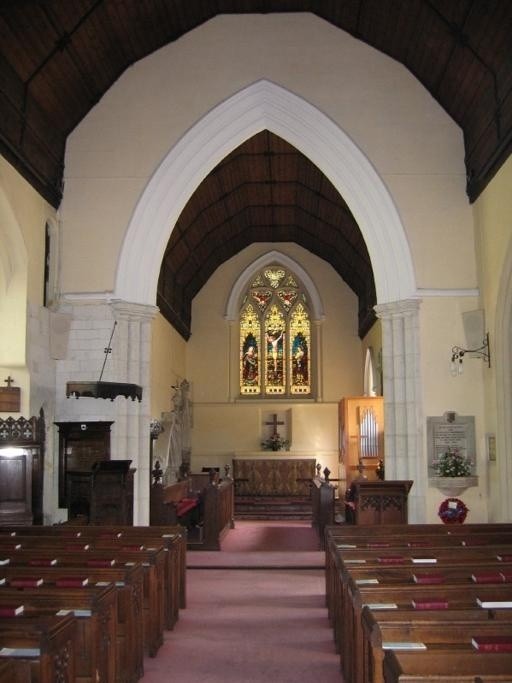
[0,524,189,683]
[324,522,512,683]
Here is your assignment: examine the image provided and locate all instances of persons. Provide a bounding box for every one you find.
[161,378,195,485]
[266,330,283,370]
[243,345,258,385]
[294,345,305,382]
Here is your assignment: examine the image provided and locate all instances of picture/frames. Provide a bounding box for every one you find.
[487,436,497,462]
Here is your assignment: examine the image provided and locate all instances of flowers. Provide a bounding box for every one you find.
[262,433,290,452]
[430,446,475,476]
[375,456,385,480]
[439,498,469,523]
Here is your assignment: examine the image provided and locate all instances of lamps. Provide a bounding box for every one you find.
[449,332,491,376]
